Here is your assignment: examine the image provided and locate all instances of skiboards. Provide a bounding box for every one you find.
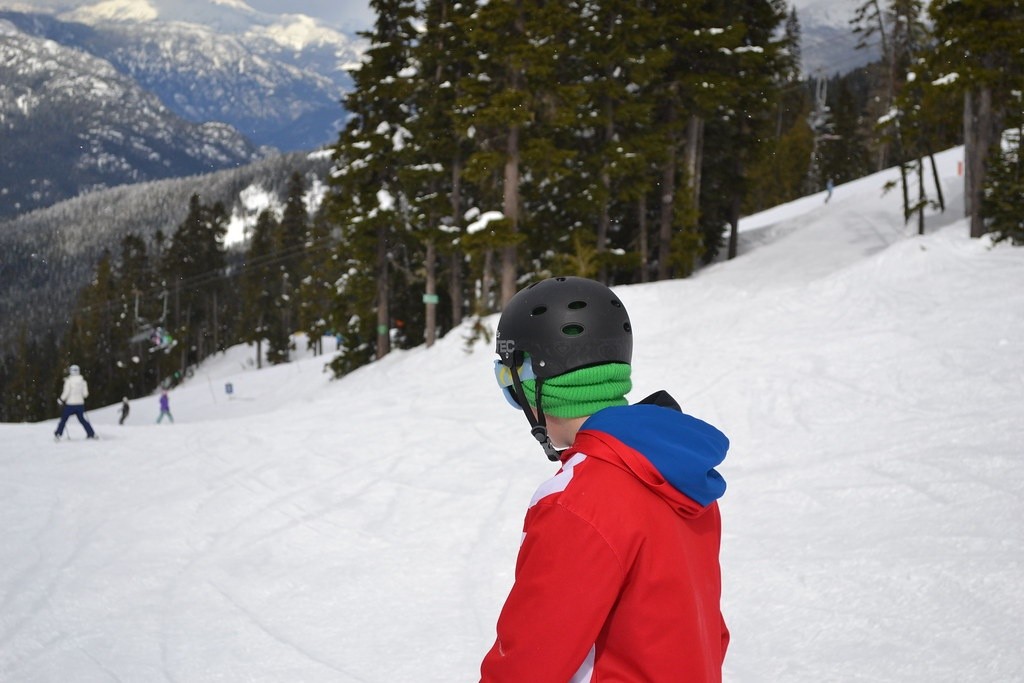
[51,432,115,444]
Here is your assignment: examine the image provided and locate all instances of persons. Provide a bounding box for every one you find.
[119,396,129,424]
[156,392,173,424]
[478,276,729,683]
[54,365,95,439]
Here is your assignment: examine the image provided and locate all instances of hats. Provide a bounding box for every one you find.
[69,365,80,376]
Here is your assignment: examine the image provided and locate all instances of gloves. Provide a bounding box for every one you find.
[57,398,64,408]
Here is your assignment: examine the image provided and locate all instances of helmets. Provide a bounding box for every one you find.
[496,275,632,387]
